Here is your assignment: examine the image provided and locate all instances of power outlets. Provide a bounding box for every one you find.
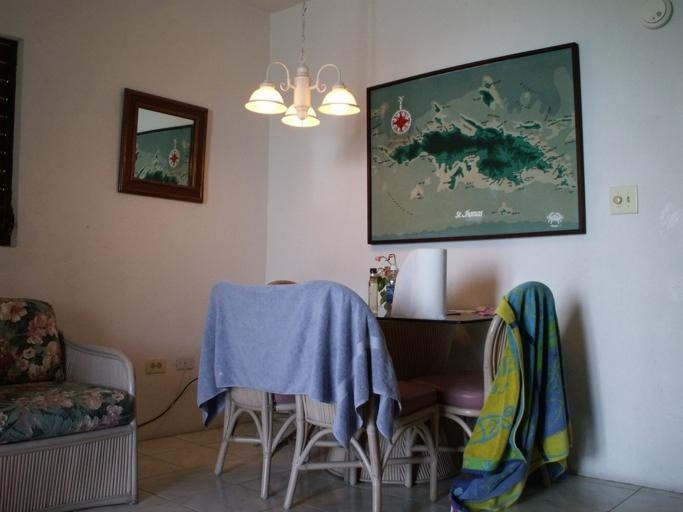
[145,357,167,374]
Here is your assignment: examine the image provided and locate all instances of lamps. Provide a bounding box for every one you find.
[243,1,361,130]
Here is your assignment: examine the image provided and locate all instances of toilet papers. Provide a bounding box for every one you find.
[388,246,449,320]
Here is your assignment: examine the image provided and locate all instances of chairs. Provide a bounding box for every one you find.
[0,296,137,510]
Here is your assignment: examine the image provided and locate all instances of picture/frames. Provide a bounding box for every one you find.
[116,85,209,204]
[364,42,585,246]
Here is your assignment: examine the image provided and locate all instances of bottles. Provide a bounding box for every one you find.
[385,264,397,315]
[367,269,377,315]
